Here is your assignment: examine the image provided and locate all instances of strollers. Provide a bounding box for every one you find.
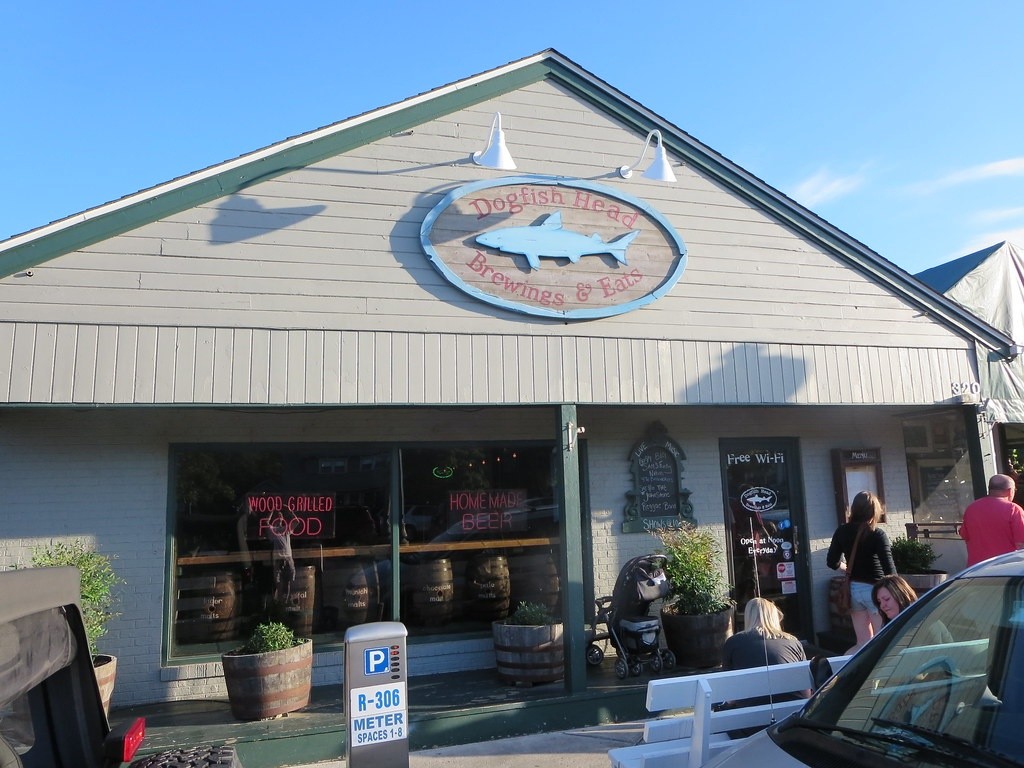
[586,553,677,679]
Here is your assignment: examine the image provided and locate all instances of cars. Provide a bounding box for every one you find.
[750,488,791,537]
[697,548,1023,768]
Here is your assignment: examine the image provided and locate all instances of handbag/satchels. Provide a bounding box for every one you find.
[828,575,851,616]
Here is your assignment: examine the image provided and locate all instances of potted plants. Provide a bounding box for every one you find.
[221,621,313,721]
[890,533,948,598]
[8,536,128,720]
[491,599,565,683]
[642,513,735,668]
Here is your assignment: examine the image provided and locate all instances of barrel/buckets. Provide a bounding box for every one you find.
[412,556,456,627]
[520,550,562,611]
[321,558,368,626]
[464,555,511,622]
[269,563,316,641]
[179,571,237,642]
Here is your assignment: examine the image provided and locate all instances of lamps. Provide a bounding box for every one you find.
[472,111,517,170]
[619,128,677,183]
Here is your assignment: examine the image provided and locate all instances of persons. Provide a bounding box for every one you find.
[720,597,811,740]
[871,576,953,647]
[959,474,1024,567]
[826,492,899,644]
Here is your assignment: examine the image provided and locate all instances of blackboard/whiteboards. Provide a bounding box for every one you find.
[916,459,963,522]
[620,419,699,534]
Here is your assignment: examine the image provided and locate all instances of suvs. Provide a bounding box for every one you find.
[376,503,443,542]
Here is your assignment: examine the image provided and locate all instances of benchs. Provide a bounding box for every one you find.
[607,638,989,768]
